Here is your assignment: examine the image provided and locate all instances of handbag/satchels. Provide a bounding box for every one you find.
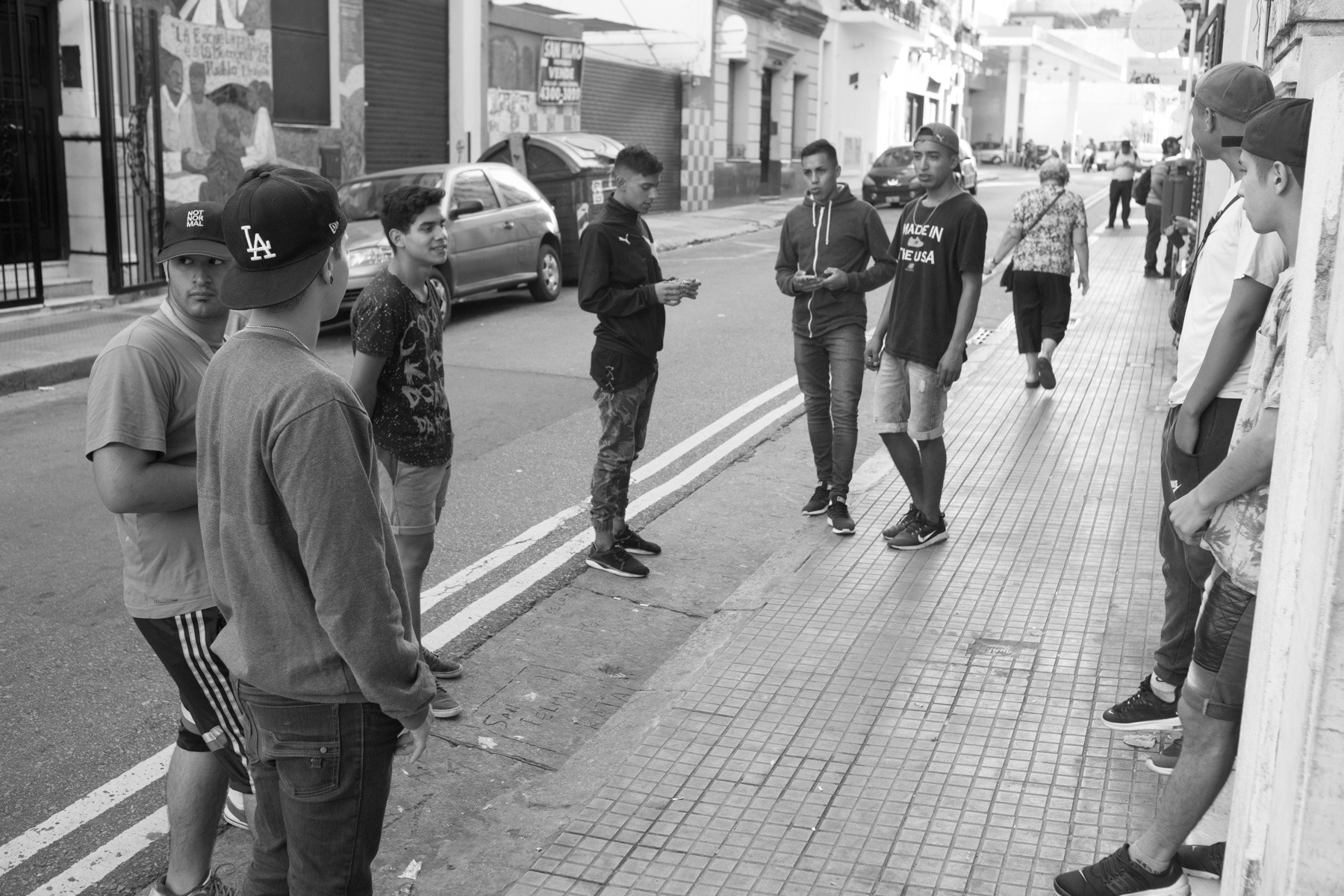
[1000,258,1014,293]
[1169,268,1191,334]
[1133,165,1151,205]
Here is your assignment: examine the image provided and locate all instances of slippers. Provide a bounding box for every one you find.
[1038,358,1057,390]
[1024,372,1041,389]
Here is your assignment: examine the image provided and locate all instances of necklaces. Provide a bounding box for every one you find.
[912,186,957,228]
[247,325,309,351]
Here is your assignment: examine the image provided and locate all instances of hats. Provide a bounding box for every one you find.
[1195,61,1275,122]
[913,123,962,174]
[1220,98,1314,168]
[1161,135,1183,154]
[217,167,347,310]
[156,198,234,264]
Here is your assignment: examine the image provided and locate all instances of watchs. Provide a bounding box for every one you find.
[991,257,998,265]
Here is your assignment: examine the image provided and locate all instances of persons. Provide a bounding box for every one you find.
[1104,61,1276,775]
[863,123,988,550]
[775,139,896,535]
[579,143,701,577]
[1023,140,1070,171]
[982,157,1089,389]
[1145,136,1183,277]
[195,162,436,896]
[1053,98,1315,896]
[1081,138,1096,173]
[1105,140,1142,228]
[345,185,464,719]
[86,200,257,896]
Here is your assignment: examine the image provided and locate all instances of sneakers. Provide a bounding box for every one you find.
[1177,841,1228,881]
[801,479,833,516]
[221,795,250,831]
[149,864,243,896]
[1053,844,1191,896]
[427,678,463,717]
[882,503,919,540]
[615,524,661,555]
[586,540,650,578]
[1101,673,1183,731]
[1146,735,1184,776]
[827,491,856,534]
[421,646,464,678]
[888,512,949,550]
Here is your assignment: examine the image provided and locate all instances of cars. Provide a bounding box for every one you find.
[862,140,979,207]
[1094,140,1135,172]
[972,140,1007,165]
[314,161,563,329]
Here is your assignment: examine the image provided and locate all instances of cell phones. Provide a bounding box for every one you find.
[801,275,826,281]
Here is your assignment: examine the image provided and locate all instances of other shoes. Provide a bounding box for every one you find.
[1163,266,1180,279]
[1143,265,1163,279]
